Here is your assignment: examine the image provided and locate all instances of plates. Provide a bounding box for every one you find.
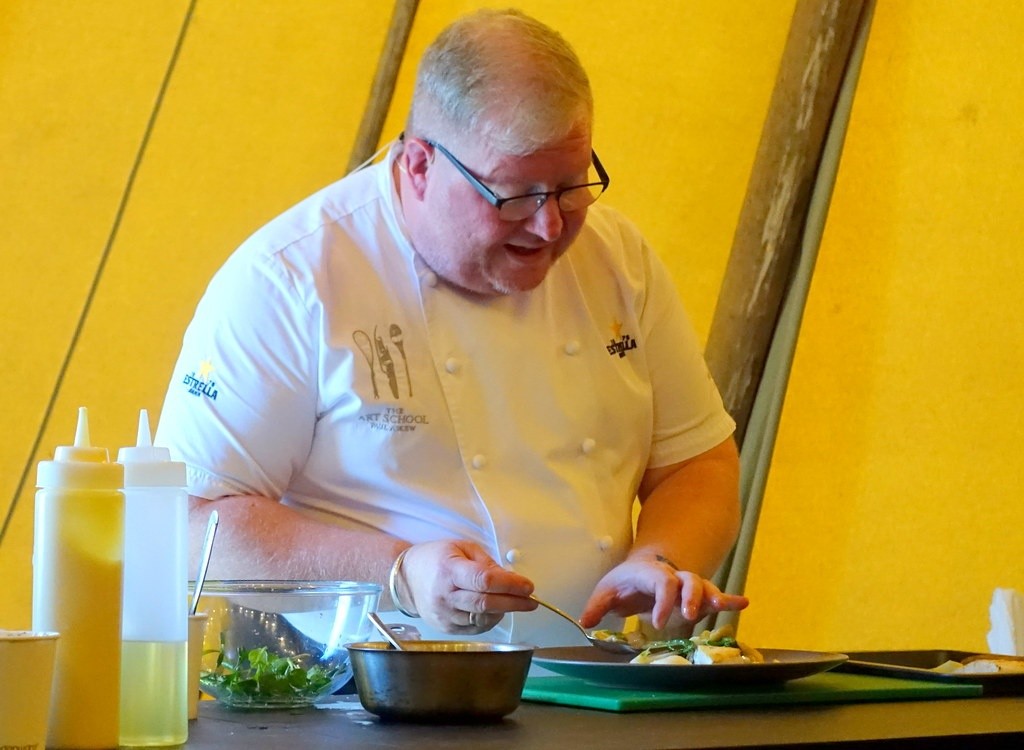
[529,644,849,694]
[826,649,1024,696]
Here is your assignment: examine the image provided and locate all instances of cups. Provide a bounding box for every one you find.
[186,612,210,722]
[0,630,61,750]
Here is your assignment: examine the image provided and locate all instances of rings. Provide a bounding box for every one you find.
[469,612,475,625]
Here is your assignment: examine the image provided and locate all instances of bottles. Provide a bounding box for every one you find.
[28,406,125,750]
[114,408,192,749]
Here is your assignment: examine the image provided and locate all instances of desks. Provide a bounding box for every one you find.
[164,653,1024,750]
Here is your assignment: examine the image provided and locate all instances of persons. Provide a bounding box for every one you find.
[154,9,749,694]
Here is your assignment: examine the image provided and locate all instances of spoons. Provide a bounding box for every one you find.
[528,593,633,655]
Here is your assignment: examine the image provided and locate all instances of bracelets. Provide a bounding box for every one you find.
[630,553,678,569]
[389,550,419,618]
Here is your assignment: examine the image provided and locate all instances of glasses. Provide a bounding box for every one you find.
[421,138,610,220]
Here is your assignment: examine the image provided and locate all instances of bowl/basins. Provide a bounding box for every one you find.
[343,640,541,724]
[187,579,386,706]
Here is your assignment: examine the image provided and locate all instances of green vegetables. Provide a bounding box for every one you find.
[201,630,337,702]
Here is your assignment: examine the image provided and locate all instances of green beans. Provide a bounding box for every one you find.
[661,634,736,654]
